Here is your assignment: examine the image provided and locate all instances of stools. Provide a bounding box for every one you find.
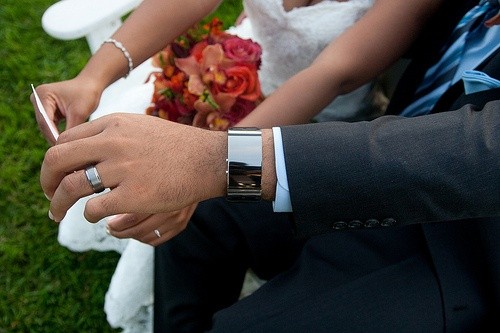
[42,0,142,55]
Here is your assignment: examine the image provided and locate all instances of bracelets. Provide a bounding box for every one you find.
[225,126,262,205]
[103,39,133,79]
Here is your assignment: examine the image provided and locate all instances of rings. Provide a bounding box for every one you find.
[153,230,161,237]
[84,165,105,194]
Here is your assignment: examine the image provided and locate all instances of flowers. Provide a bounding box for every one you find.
[142,18,265,131]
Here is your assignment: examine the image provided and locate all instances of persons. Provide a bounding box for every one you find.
[30,0,439,333]
[40,0,500,333]
